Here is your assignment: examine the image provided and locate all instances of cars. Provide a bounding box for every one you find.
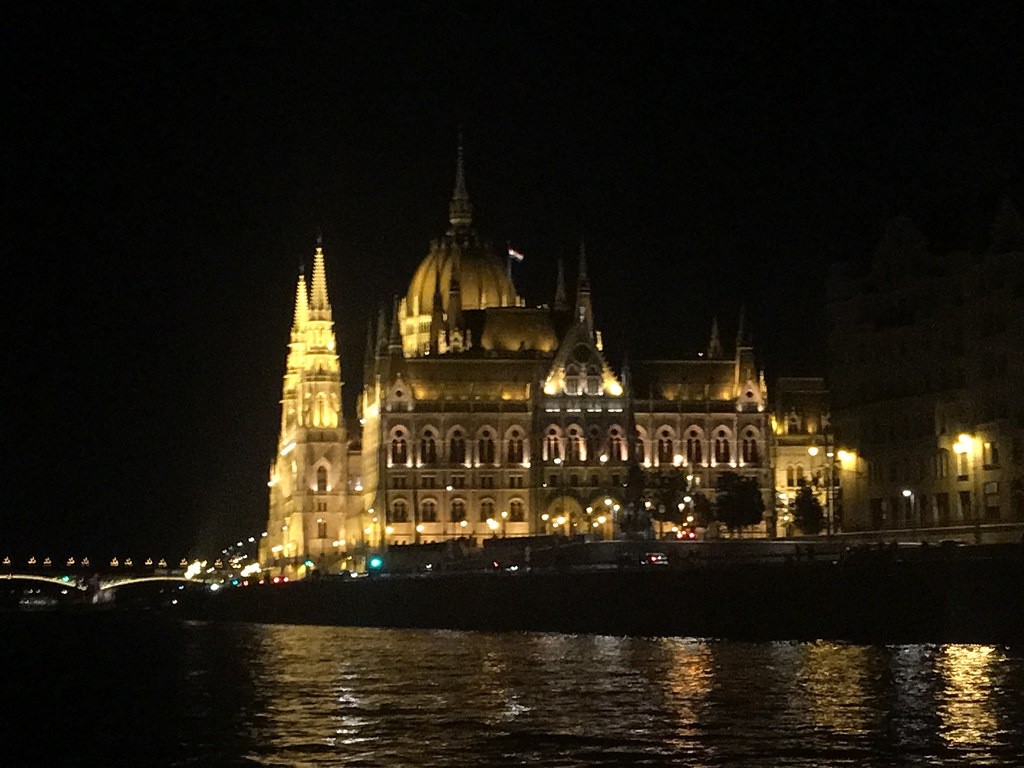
[638,551,668,566]
[339,569,358,578]
[933,540,968,546]
